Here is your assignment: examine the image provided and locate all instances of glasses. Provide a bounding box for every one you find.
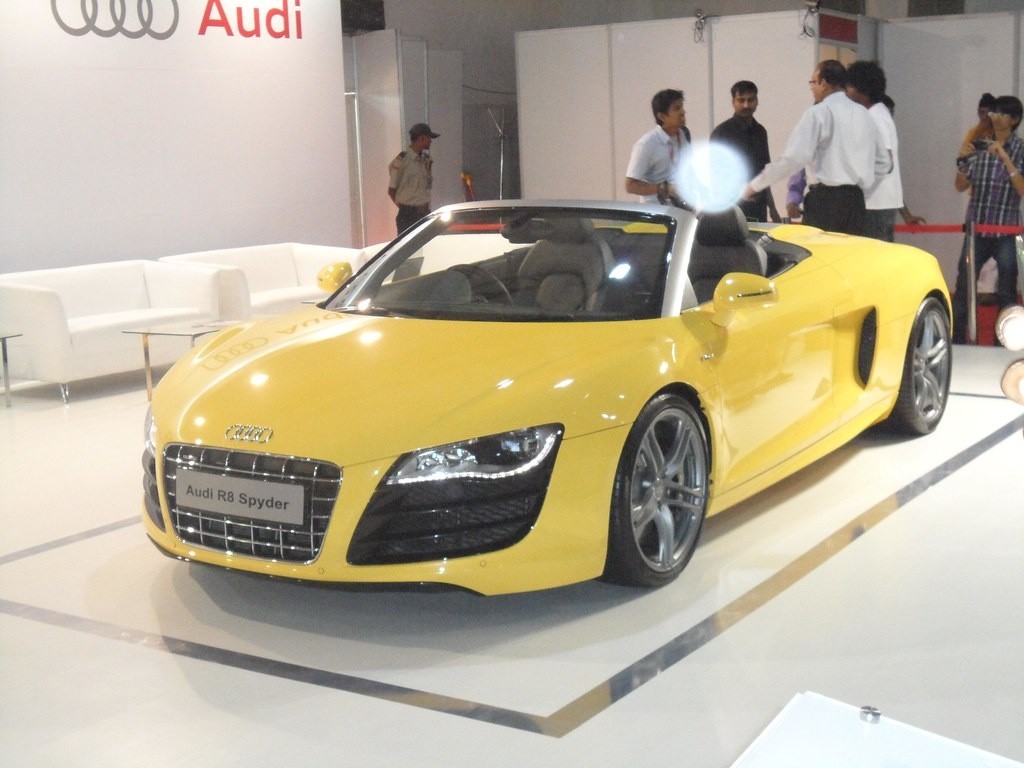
[809,80,831,86]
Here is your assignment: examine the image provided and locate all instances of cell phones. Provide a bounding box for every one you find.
[973,140,995,152]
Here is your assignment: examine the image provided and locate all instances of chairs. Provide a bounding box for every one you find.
[364,234,534,284]
[516,216,615,312]
[688,206,767,303]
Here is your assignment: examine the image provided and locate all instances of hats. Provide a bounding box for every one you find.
[409,123,440,138]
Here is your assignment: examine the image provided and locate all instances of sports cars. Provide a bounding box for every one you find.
[140,186,958,602]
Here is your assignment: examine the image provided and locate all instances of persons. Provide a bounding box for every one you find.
[950,95,1024,347]
[954,91,997,160]
[739,60,888,237]
[709,80,782,223]
[387,123,441,237]
[623,88,693,207]
[844,61,927,243]
[785,168,808,220]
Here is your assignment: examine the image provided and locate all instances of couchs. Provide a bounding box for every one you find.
[0,243,362,408]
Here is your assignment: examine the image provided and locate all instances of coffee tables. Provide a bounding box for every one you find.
[123,319,230,403]
